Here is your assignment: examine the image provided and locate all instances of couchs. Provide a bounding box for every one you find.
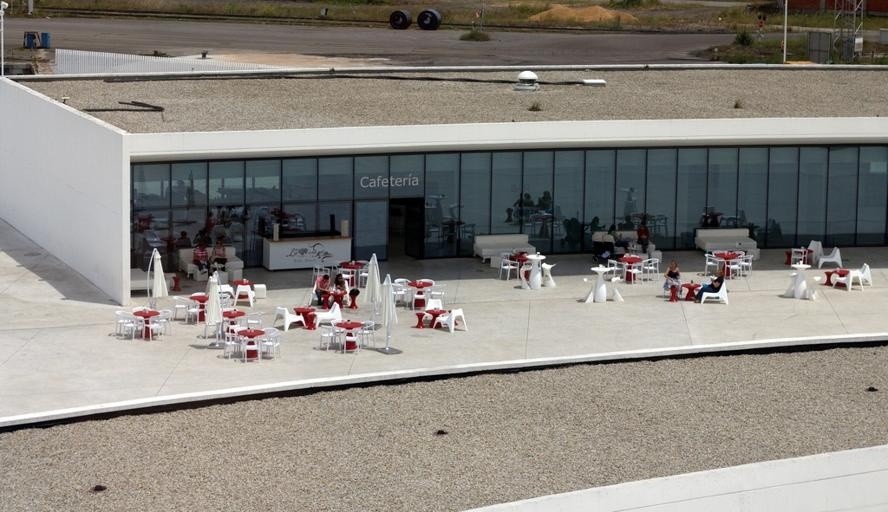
[178,246,244,281]
[472,234,537,264]
[696,229,758,256]
[591,231,655,259]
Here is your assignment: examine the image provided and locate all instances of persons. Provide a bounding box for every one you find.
[637,221,649,253]
[175,208,245,247]
[499,191,553,222]
[662,259,681,302]
[192,244,210,274]
[329,273,349,306]
[689,270,724,303]
[314,273,330,305]
[208,240,228,272]
[588,215,633,249]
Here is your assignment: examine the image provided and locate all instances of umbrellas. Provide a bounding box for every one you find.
[204,275,224,345]
[146,249,168,314]
[380,272,397,348]
[364,253,382,326]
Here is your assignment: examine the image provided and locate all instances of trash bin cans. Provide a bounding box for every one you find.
[144,240,167,273]
[421,222,443,258]
[459,223,475,256]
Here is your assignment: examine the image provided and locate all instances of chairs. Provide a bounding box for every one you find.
[133,202,305,268]
[425,191,475,246]
[615,187,667,244]
[700,204,783,244]
[491,239,752,304]
[778,240,873,301]
[504,190,606,251]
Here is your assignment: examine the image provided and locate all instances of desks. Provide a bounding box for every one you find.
[262,230,352,271]
